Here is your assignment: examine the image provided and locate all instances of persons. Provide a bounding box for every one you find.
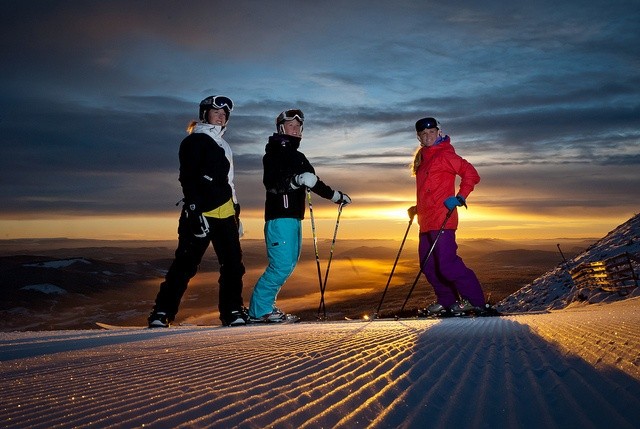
[149,95,244,328]
[408,116,490,313]
[252,109,352,323]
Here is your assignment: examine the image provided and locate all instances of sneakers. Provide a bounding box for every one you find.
[148,311,170,327]
[223,310,246,326]
[452,301,491,317]
[417,303,458,318]
[247,310,286,322]
[283,314,300,323]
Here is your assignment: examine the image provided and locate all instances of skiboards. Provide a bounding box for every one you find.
[179,314,301,327]
[374,311,548,321]
[95,314,287,332]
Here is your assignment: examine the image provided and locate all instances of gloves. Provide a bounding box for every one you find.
[444,195,466,210]
[185,203,209,238]
[332,189,352,207]
[235,204,243,238]
[290,172,318,189]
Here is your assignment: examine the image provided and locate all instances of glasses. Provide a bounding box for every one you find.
[278,109,304,122]
[200,95,234,111]
[416,118,442,131]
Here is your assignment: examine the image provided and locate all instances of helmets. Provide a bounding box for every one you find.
[416,118,442,142]
[200,95,234,127]
[276,110,305,135]
